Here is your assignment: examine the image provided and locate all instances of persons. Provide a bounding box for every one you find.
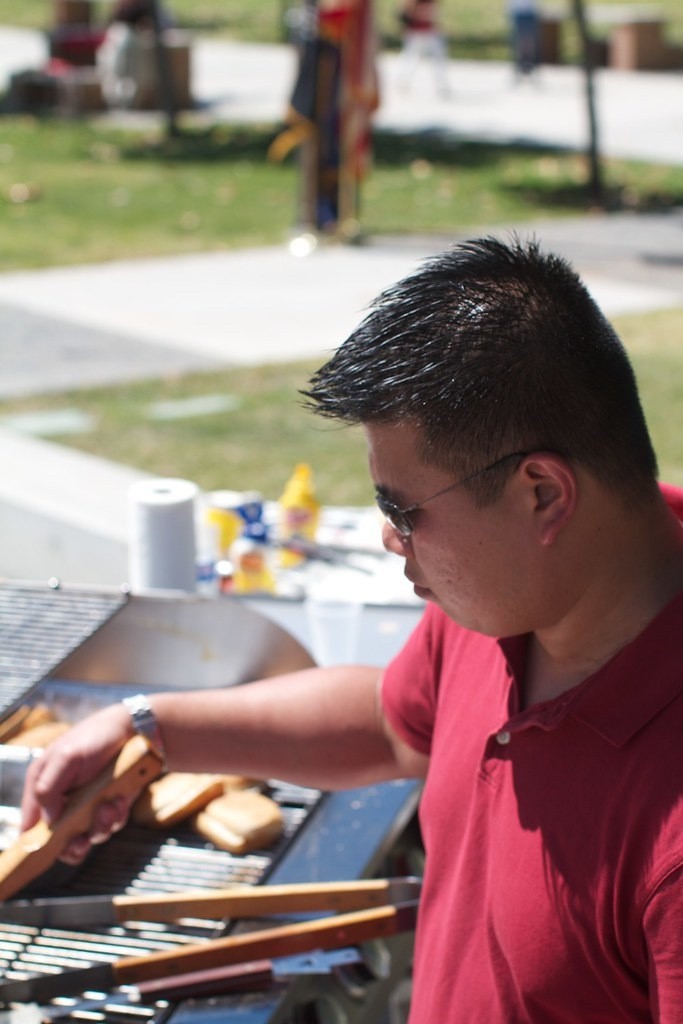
[45,0,543,109]
[20,234,683,1024]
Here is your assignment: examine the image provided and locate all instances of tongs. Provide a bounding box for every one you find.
[0,876,424,1004]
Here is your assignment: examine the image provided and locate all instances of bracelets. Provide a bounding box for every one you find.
[122,693,170,776]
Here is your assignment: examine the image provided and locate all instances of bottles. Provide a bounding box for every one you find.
[278,465,322,570]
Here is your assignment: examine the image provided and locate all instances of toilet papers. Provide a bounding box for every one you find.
[126,476,197,591]
